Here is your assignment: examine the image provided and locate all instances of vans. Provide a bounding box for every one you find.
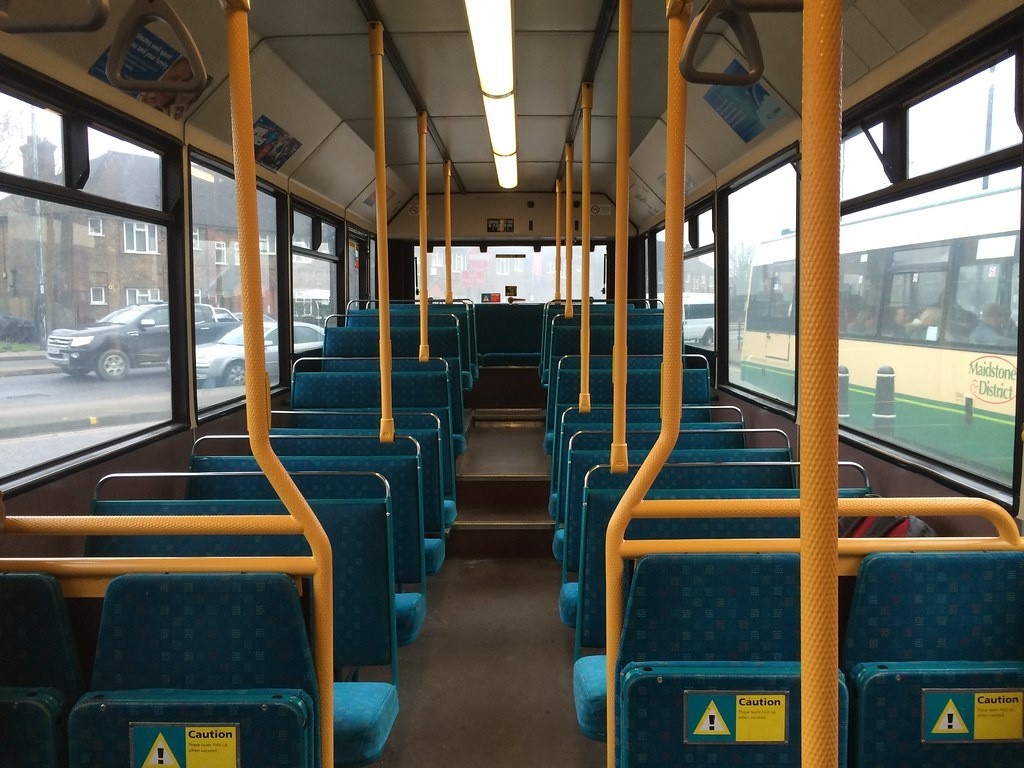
[646,290,715,347]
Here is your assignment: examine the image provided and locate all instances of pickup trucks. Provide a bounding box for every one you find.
[46,300,244,382]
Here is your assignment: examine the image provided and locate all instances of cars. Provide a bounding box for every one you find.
[165,319,325,387]
[213,311,276,322]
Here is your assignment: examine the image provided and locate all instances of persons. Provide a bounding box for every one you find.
[839,283,1018,348]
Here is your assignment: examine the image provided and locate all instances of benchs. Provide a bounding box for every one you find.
[52,302,943,764]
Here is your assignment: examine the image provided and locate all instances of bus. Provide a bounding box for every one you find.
[739,185,1023,491]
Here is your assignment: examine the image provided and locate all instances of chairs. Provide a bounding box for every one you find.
[838,551,1024,768]
[0,571,80,768]
[64,572,323,768]
[615,552,850,768]
